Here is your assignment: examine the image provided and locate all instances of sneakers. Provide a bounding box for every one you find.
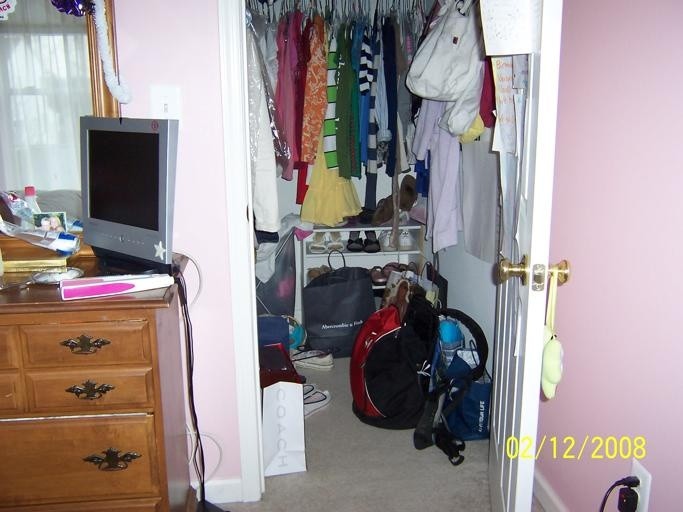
[303,383,332,420]
[290,348,334,371]
[371,262,418,285]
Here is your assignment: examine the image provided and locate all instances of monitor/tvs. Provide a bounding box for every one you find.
[78,115,180,276]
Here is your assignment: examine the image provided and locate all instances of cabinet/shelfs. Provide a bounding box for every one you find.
[0,251,192,512]
[288,225,429,338]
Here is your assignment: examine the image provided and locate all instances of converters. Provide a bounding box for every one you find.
[617,488,638,512]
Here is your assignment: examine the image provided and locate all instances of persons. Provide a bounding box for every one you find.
[36,216,64,232]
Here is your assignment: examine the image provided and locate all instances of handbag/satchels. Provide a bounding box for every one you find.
[259,343,307,477]
[381,271,439,327]
[303,250,377,358]
[405,0,485,103]
[443,339,492,442]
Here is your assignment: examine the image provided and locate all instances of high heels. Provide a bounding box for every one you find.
[308,265,331,282]
[308,229,413,253]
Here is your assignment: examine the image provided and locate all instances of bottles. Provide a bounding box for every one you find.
[19,185,42,231]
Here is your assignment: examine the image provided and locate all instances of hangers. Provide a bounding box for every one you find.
[239,1,436,27]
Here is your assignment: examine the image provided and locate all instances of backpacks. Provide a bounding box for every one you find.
[350,306,489,430]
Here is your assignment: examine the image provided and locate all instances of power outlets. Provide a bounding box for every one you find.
[627,457,652,511]
